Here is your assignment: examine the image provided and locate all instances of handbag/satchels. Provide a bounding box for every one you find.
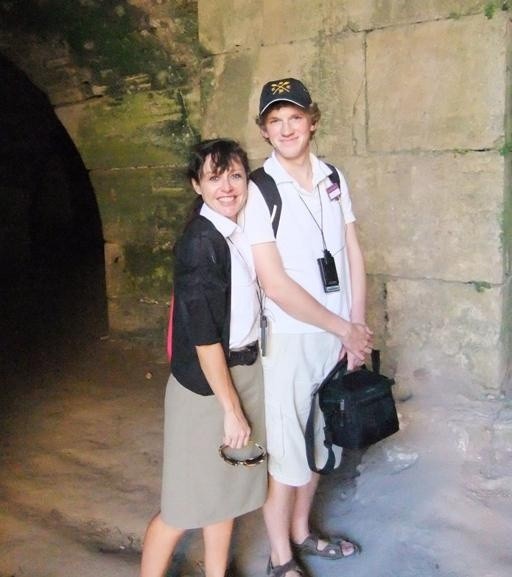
[304,348,400,476]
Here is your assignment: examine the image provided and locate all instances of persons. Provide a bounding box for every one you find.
[137,137,269,576]
[236,76,373,576]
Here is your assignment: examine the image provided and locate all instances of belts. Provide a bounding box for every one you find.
[228,340,260,369]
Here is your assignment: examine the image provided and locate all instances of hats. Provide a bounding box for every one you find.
[258,77,312,117]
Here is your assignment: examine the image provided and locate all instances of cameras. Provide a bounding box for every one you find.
[318,254,340,293]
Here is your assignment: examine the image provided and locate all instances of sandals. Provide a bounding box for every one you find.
[290,530,361,560]
[265,555,307,577]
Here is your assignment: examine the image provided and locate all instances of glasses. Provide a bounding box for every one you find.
[198,136,240,151]
[216,439,267,467]
